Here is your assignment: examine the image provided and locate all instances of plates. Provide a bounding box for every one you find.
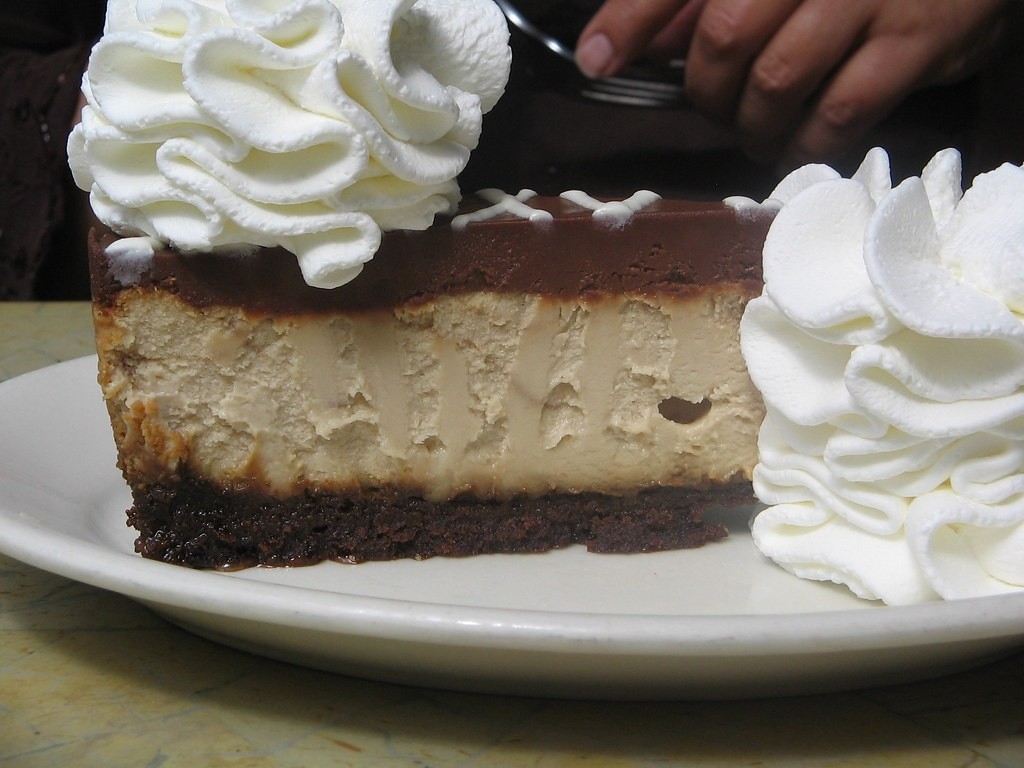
[0,354,1023,703]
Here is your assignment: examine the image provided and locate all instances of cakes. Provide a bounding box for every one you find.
[67,0,1024,606]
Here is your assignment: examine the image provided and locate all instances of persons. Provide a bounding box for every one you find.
[572,0,1024,167]
